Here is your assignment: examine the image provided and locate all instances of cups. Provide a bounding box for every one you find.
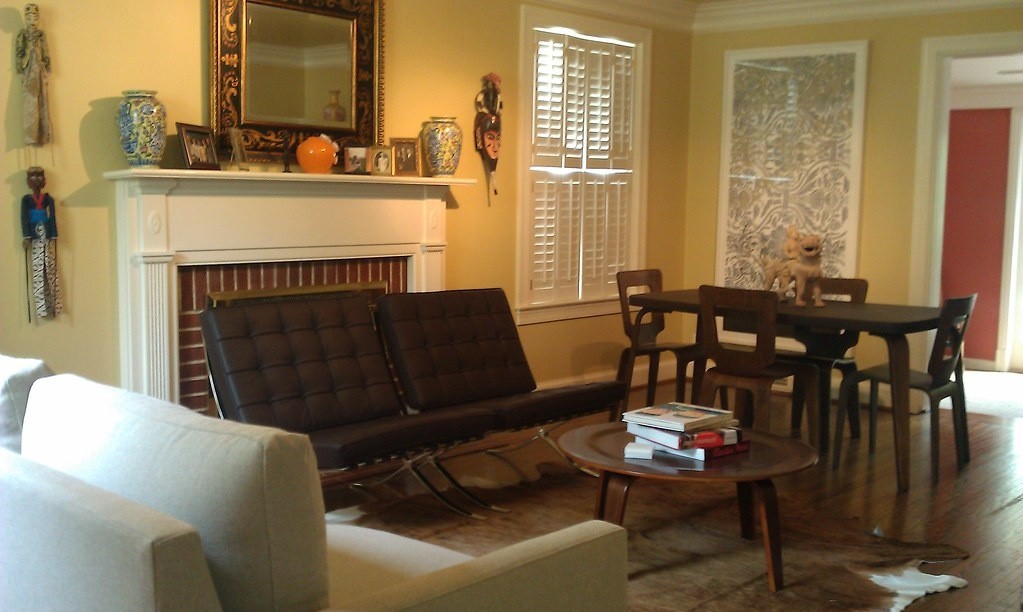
[344,146,369,175]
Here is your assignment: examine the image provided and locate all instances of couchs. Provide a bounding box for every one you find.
[0,355,626,612]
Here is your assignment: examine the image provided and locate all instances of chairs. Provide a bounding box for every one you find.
[770,276,870,454]
[834,292,979,488]
[694,285,821,475]
[609,270,729,420]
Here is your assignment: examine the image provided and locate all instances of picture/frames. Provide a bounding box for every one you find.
[175,121,222,170]
[389,137,422,177]
[227,128,251,172]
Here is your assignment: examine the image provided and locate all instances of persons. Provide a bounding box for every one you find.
[21,166,63,321]
[15,3,52,145]
[397,148,412,169]
[378,153,388,172]
[186,136,214,163]
[352,155,360,165]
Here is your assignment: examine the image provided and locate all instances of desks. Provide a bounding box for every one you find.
[557,421,819,594]
[629,287,971,492]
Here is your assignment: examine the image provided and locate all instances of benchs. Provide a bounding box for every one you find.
[370,288,624,513]
[200,295,490,522]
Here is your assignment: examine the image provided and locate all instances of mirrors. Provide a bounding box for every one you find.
[207,0,384,166]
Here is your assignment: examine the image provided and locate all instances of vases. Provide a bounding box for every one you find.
[423,116,464,179]
[322,90,346,123]
[114,89,168,168]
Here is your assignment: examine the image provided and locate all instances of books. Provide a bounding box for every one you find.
[622,401,751,460]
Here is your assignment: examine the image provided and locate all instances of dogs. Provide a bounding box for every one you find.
[760,233,826,307]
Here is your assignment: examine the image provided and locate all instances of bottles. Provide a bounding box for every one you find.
[323,90,346,121]
[117,90,167,170]
[297,134,339,173]
[423,117,463,178]
